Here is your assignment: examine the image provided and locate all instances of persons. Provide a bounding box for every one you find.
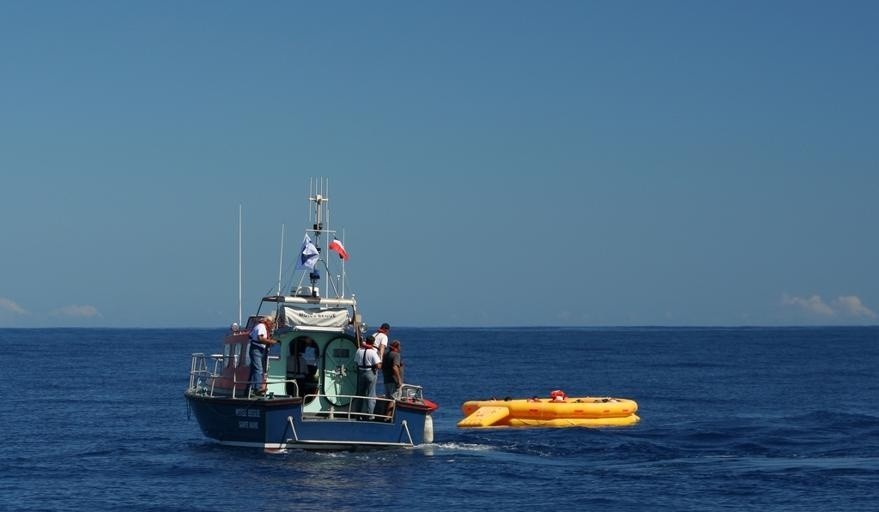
[243,316,277,398]
[350,335,382,420]
[383,340,402,422]
[504,397,512,401]
[372,323,389,361]
[550,385,565,401]
[298,338,318,383]
[527,396,540,402]
[491,397,497,401]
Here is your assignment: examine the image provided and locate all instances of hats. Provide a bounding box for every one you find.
[381,323,390,329]
[390,340,400,350]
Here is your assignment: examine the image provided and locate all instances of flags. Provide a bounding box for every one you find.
[329,237,349,261]
[295,233,320,270]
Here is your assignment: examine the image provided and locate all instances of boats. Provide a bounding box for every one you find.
[457,389,644,430]
[182,177,438,457]
